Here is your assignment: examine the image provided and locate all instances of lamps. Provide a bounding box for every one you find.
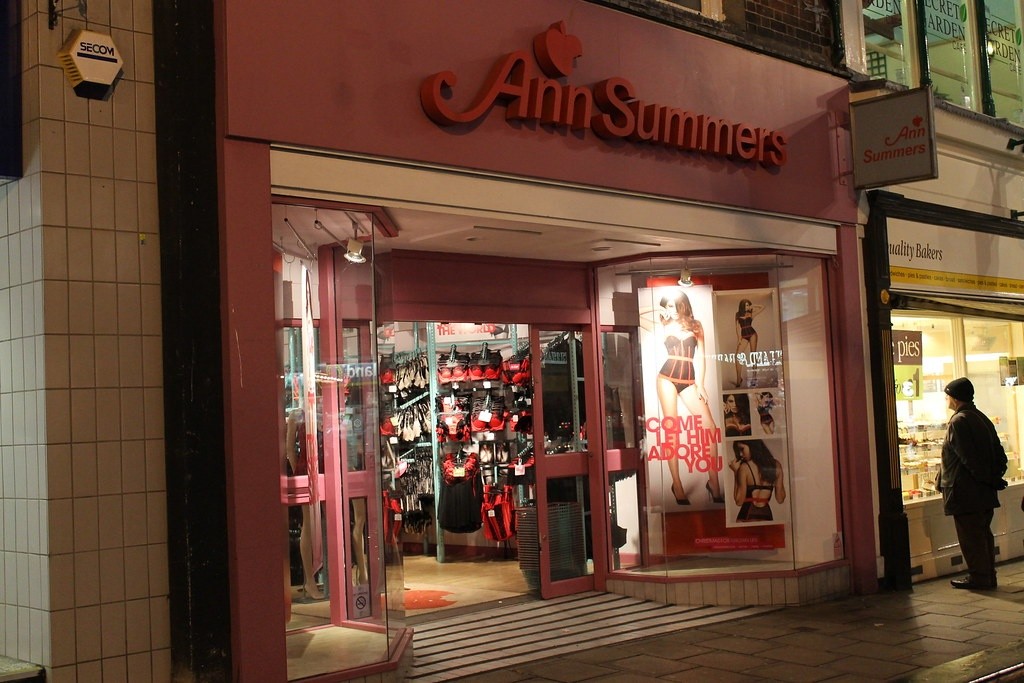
[342,223,368,265]
[677,256,694,287]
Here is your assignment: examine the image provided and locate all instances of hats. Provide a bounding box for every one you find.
[944,378,974,401]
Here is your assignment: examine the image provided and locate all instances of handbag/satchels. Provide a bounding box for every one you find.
[935,467,943,492]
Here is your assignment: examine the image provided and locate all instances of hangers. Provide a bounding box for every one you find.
[394,333,582,513]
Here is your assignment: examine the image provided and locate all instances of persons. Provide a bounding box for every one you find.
[347,435,366,584]
[728,439,786,524]
[287,374,327,600]
[938,378,1009,592]
[754,391,781,436]
[723,393,752,436]
[898,433,916,445]
[734,299,766,388]
[658,288,723,505]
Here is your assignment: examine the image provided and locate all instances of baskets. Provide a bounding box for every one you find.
[515,501,586,590]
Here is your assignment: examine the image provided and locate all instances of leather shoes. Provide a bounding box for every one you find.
[951,573,997,589]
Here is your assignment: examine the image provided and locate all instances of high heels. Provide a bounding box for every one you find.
[671,484,690,504]
[706,480,724,502]
